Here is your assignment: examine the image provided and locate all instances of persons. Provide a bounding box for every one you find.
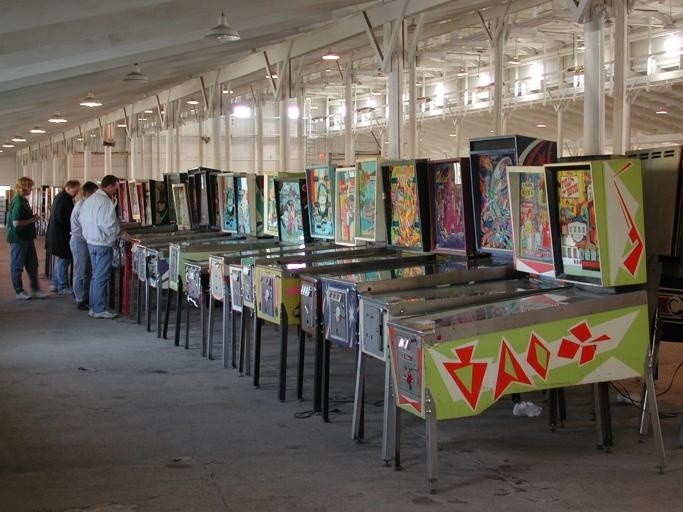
[44,181,81,296]
[69,181,99,311]
[5,176,47,301]
[77,174,121,319]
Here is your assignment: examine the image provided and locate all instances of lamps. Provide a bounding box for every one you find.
[120,63,149,84]
[9,88,102,143]
[200,8,240,45]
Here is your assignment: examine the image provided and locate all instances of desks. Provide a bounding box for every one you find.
[294,257,445,410]
[119,222,403,401]
[384,285,664,485]
[350,281,558,462]
[322,261,523,440]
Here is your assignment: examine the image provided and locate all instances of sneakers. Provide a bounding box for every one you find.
[31,291,48,299]
[49,284,119,318]
[15,291,31,300]
